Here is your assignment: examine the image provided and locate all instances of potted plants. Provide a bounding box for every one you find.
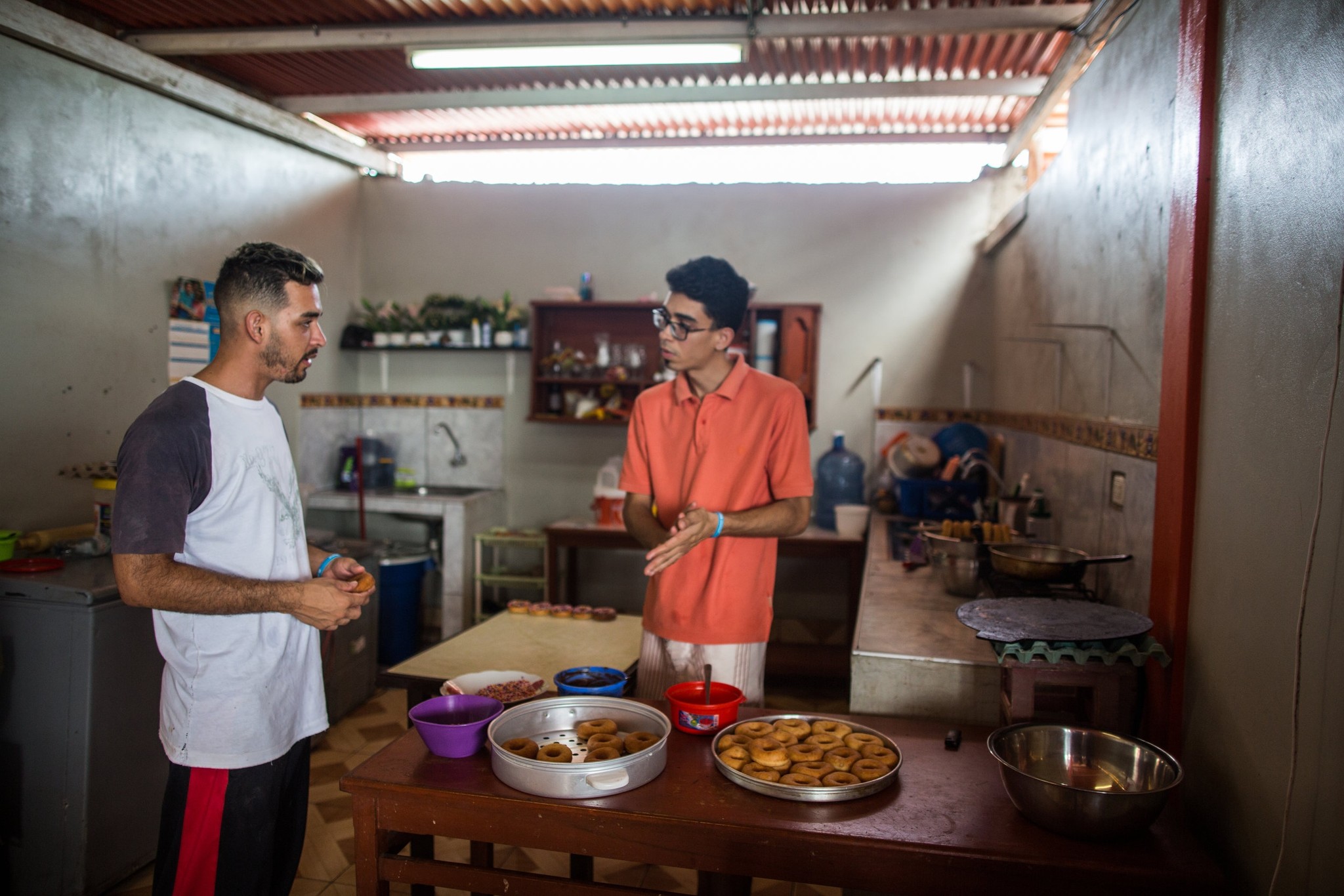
[360,294,519,347]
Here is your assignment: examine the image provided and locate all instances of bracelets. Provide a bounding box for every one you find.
[317,554,341,578]
[710,512,724,537]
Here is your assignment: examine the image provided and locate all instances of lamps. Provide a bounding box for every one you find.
[404,38,750,69]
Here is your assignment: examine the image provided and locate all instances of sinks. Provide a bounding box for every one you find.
[373,484,483,497]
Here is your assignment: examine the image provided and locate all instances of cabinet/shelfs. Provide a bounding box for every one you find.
[528,294,758,429]
[474,533,567,623]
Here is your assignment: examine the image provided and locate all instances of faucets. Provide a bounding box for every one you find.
[433,421,467,469]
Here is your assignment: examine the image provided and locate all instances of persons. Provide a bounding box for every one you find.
[172,281,204,319]
[110,241,374,896]
[617,256,813,709]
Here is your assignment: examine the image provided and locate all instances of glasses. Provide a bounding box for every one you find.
[651,305,713,341]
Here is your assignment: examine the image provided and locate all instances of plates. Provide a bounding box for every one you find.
[440,670,550,704]
[711,713,903,802]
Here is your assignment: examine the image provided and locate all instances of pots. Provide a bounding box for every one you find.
[915,520,1020,591]
[989,541,1135,579]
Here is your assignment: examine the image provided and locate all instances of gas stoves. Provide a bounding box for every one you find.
[986,571,1092,600]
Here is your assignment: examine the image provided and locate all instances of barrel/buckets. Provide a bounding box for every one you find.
[996,493,1026,534]
[588,483,627,527]
[890,477,973,527]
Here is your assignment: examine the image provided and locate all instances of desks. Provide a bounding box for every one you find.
[337,671,1243,895]
[389,608,650,729]
[549,515,629,603]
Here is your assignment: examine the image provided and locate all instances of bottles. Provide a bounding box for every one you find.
[92,478,118,548]
[813,430,867,531]
[1025,490,1052,539]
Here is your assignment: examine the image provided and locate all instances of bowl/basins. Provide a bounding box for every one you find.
[661,680,748,735]
[1,534,19,561]
[835,503,869,537]
[553,666,629,696]
[407,694,505,758]
[985,719,1185,832]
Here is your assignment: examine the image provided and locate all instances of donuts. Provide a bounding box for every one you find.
[940,519,1011,542]
[500,719,660,763]
[348,572,375,593]
[508,600,617,622]
[718,719,897,787]
